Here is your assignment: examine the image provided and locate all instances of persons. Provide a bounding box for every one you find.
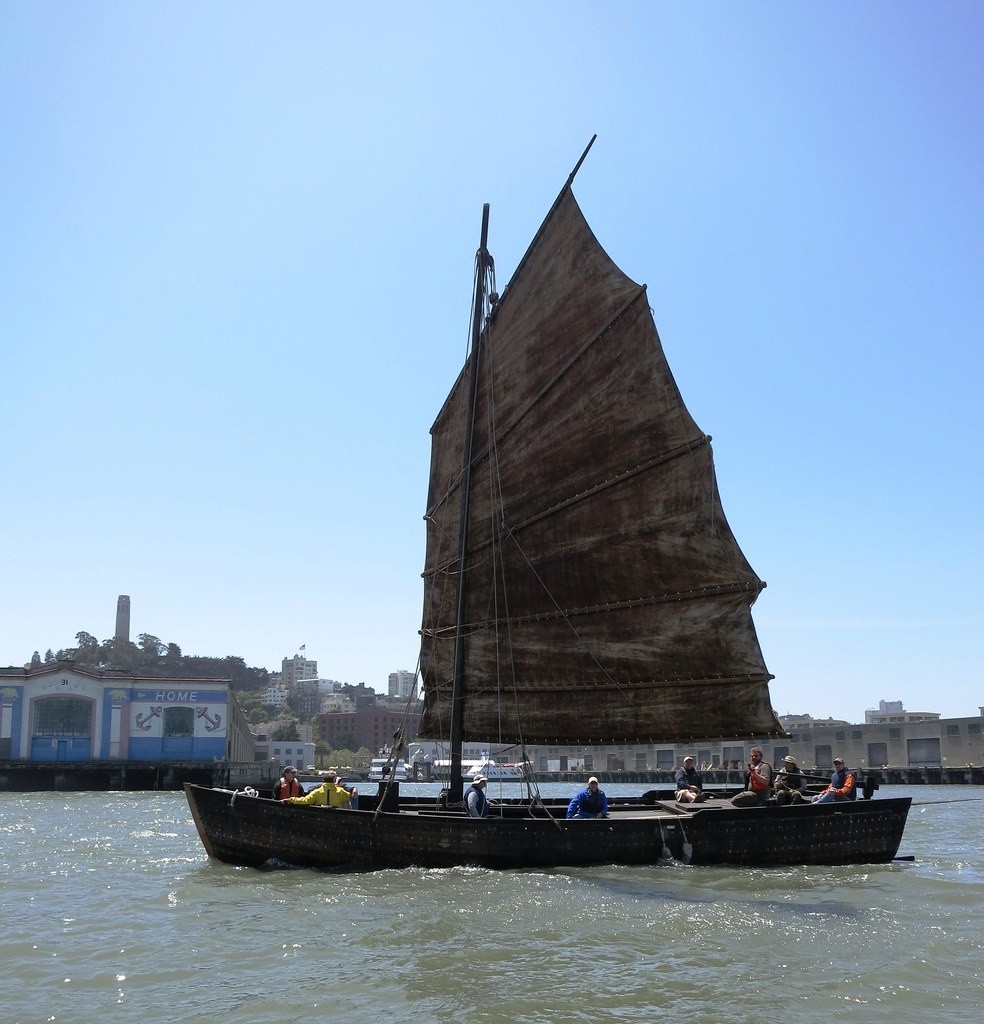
[280,770,358,809]
[273,765,304,800]
[811,757,857,803]
[731,747,773,807]
[567,777,607,819]
[774,755,807,805]
[464,774,489,818]
[675,756,706,803]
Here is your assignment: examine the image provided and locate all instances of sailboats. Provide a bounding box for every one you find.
[182,132,914,871]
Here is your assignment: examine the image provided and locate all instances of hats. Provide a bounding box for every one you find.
[781,756,798,766]
[283,765,299,774]
[588,777,598,785]
[471,774,489,785]
[683,757,693,763]
[833,757,844,763]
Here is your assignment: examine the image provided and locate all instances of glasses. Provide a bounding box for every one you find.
[751,754,758,758]
[834,762,842,765]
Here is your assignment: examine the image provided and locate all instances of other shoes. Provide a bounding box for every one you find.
[695,795,705,803]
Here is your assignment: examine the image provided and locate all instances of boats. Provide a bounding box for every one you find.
[460,761,526,783]
